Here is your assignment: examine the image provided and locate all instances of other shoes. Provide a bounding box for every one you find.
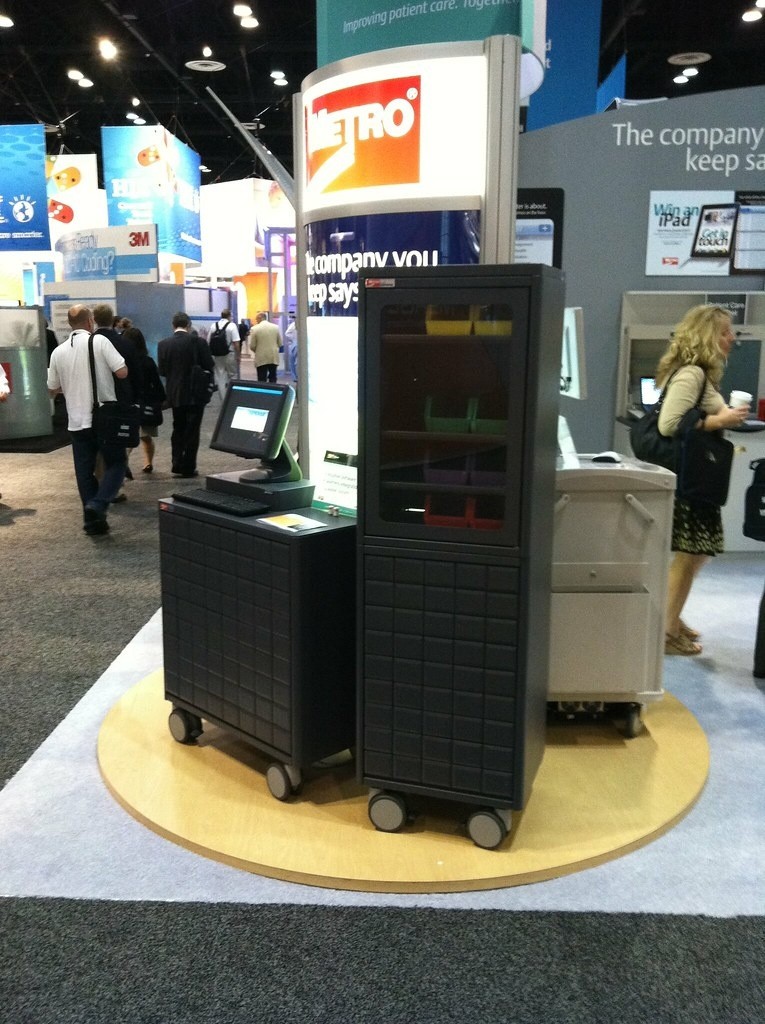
[142,464,153,471]
[86,502,107,521]
[172,466,199,478]
[85,524,110,535]
[125,464,134,481]
[109,493,126,501]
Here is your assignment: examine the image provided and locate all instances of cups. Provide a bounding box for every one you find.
[728,390,753,427]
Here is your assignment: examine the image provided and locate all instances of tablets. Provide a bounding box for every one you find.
[690,203,741,259]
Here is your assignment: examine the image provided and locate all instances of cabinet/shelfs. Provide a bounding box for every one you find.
[612,289,765,555]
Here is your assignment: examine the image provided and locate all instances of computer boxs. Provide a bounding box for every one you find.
[206,469,315,511]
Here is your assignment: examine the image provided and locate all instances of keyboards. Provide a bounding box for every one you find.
[171,488,272,518]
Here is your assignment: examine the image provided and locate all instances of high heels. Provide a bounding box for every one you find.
[679,620,698,640]
[665,630,702,656]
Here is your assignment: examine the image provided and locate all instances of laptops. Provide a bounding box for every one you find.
[640,376,662,412]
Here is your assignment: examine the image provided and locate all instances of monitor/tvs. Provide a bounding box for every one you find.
[560,307,587,400]
[210,379,302,484]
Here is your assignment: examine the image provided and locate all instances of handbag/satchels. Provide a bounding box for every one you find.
[627,364,706,473]
[91,401,140,449]
[742,458,765,541]
[137,394,163,426]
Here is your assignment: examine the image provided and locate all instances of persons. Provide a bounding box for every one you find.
[0,364,10,498]
[249,313,282,384]
[207,311,241,400]
[655,305,750,655]
[93,304,167,502]
[47,304,127,535]
[239,319,250,353]
[158,312,215,477]
[286,322,298,382]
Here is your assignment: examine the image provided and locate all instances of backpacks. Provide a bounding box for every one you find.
[210,321,231,355]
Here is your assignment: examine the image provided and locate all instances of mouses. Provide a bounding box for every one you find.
[592,451,622,464]
